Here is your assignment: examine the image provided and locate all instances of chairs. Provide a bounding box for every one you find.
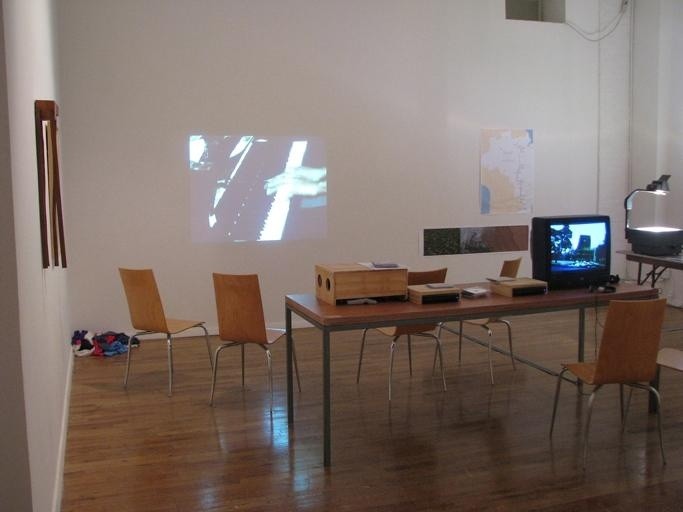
[119,267,217,399]
[618,346,683,442]
[353,264,450,403]
[207,271,303,418]
[431,260,524,388]
[551,296,669,470]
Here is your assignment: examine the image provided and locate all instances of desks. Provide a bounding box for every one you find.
[615,243,683,287]
[279,273,666,470]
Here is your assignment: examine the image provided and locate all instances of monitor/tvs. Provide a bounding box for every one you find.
[530,214,611,291]
